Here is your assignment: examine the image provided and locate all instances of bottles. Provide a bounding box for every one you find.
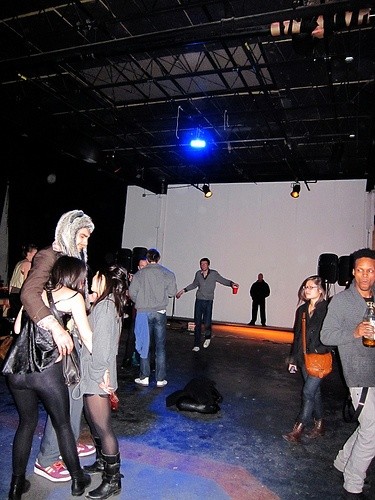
[362,302,375,347]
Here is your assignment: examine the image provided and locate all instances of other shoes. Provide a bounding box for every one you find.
[120,357,133,369]
[203,339,211,348]
[192,347,200,351]
[343,488,375,500]
[330,464,372,484]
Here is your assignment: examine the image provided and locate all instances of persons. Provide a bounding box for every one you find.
[319,247,375,500]
[129,249,181,386]
[248,273,270,326]
[176,258,239,352]
[76,263,123,500]
[2,256,91,500]
[9,244,41,332]
[282,276,327,442]
[19,209,98,483]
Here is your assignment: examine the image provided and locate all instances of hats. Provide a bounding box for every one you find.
[54,210,96,310]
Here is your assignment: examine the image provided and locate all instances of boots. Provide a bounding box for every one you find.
[81,438,105,474]
[85,451,124,500]
[282,422,304,443]
[305,419,325,438]
[69,469,91,496]
[8,474,30,500]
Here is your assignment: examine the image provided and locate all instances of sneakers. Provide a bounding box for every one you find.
[134,377,150,386]
[157,380,168,387]
[75,444,96,457]
[32,459,72,482]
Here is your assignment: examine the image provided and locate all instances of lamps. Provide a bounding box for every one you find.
[202,185,212,197]
[290,184,301,198]
[191,127,207,148]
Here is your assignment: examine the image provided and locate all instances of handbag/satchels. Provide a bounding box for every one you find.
[304,353,333,377]
[46,289,83,384]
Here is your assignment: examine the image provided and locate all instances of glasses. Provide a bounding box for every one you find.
[303,286,318,289]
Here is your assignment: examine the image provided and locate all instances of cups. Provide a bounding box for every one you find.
[232,288,238,294]
